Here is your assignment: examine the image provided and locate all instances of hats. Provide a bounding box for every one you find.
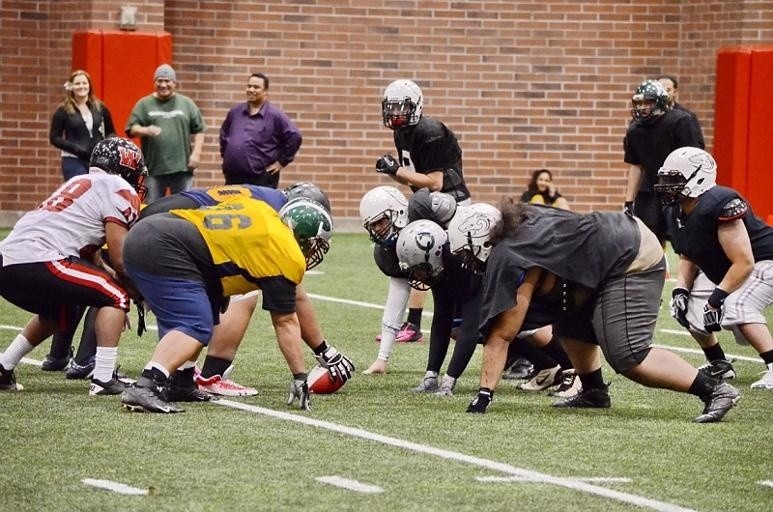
[153,64,176,81]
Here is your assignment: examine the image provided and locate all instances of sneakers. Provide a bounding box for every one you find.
[749,363,773,390]
[193,364,259,397]
[375,322,425,344]
[547,368,582,398]
[0,363,24,393]
[551,382,611,408]
[159,378,219,402]
[502,359,534,379]
[120,377,185,414]
[41,345,75,372]
[693,382,741,424]
[516,364,563,391]
[67,357,96,380]
[89,365,136,396]
[697,359,736,380]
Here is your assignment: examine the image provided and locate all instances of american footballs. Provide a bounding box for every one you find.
[307,364,346,394]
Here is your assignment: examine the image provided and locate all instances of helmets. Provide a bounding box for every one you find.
[279,197,333,272]
[382,79,423,128]
[284,182,330,212]
[396,220,450,291]
[449,203,504,275]
[631,80,669,123]
[654,146,717,207]
[90,137,149,202]
[358,185,408,245]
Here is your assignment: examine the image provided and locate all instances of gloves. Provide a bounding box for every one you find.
[312,339,356,384]
[407,370,438,393]
[375,155,401,176]
[703,286,729,334]
[465,387,494,414]
[286,372,312,412]
[670,287,691,330]
[624,201,638,224]
[434,374,456,397]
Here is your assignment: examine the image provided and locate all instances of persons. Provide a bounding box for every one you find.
[354,184,538,381]
[0,140,354,413]
[446,200,743,425]
[653,144,773,391]
[220,73,302,190]
[126,65,206,203]
[621,79,706,247]
[396,217,592,400]
[655,73,706,152]
[49,71,117,182]
[373,77,475,343]
[518,168,573,212]
